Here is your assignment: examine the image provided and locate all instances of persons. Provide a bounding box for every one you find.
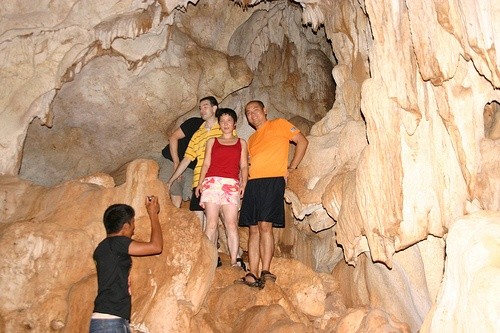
[88,195,163,333]
[240,100,309,283]
[161,118,205,208]
[194,108,248,267]
[168,96,237,267]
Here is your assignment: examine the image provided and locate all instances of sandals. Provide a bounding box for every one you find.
[216,254,276,287]
